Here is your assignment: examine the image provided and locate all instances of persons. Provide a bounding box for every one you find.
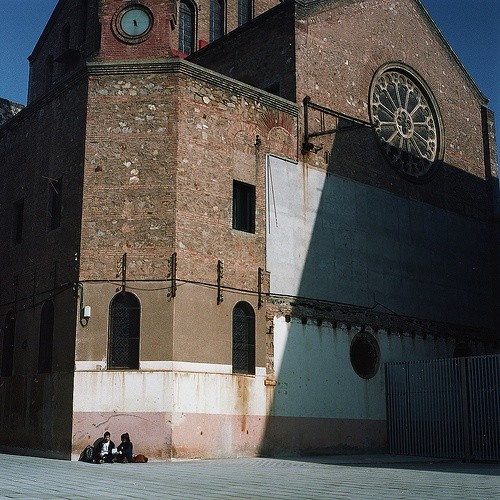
[111,433,133,463]
[89,431,115,464]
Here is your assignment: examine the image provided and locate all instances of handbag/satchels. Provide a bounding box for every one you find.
[133,454,148,463]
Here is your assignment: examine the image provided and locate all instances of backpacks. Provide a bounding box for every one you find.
[83,445,93,461]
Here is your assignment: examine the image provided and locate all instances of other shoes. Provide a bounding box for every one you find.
[111,459,115,463]
[93,459,96,463]
